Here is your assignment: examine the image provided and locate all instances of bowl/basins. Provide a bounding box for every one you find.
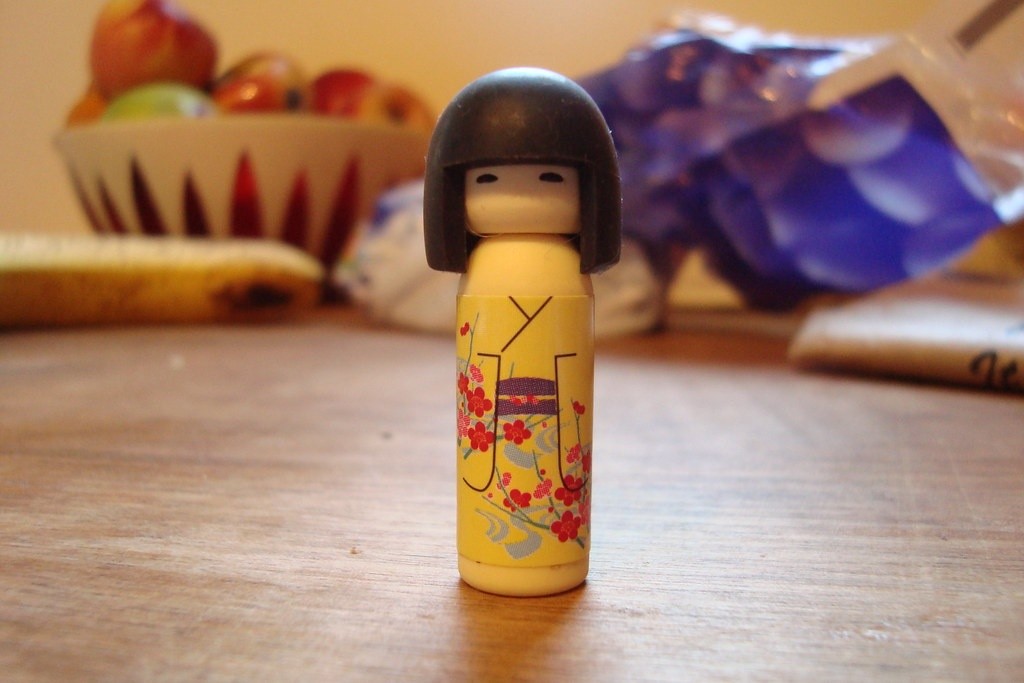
[54,119,432,298]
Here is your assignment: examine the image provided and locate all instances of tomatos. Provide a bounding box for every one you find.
[65,2,433,125]
